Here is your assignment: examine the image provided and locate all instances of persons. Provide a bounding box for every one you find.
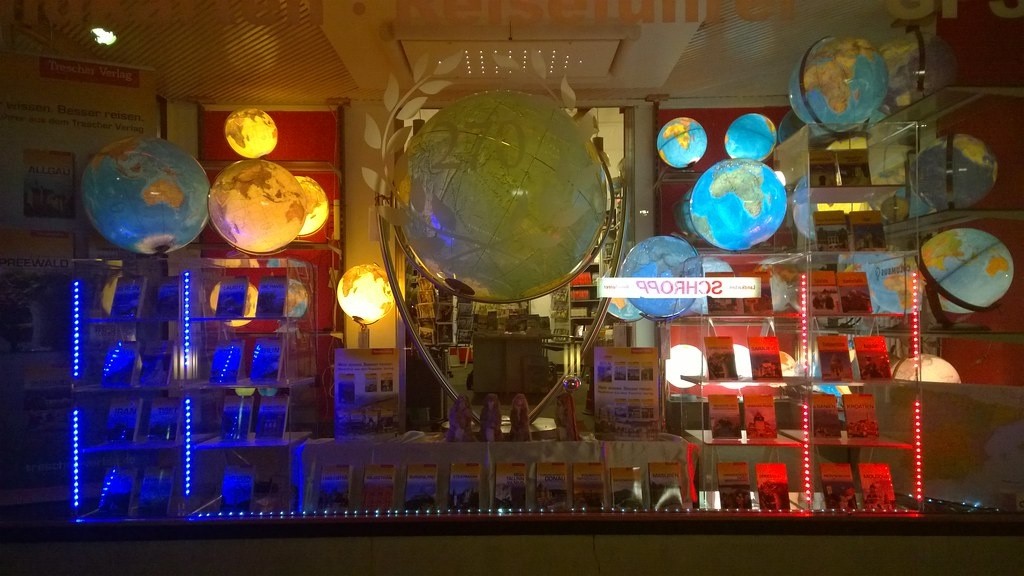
[813,293,820,309]
[823,291,835,310]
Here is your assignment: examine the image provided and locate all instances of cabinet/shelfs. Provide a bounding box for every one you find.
[568,261,600,343]
[472,334,544,407]
[70,267,319,515]
[194,100,347,438]
[679,122,923,513]
[865,84,1023,345]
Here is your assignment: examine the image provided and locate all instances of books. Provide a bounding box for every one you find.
[404,463,439,514]
[333,347,401,446]
[593,347,661,442]
[317,462,353,514]
[610,466,644,513]
[494,462,528,514]
[572,462,607,514]
[648,461,686,512]
[704,269,898,511]
[536,462,569,513]
[449,462,480,514]
[98,276,293,516]
[361,464,397,516]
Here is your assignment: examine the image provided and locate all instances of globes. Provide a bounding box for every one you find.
[374,88,617,437]
[606,234,734,321]
[683,156,817,252]
[707,338,874,424]
[78,134,211,283]
[721,111,779,160]
[223,105,281,160]
[666,345,708,400]
[889,352,962,383]
[336,260,395,349]
[654,116,709,168]
[911,225,1015,314]
[785,32,890,135]
[208,278,308,334]
[208,158,333,258]
[909,132,999,211]
